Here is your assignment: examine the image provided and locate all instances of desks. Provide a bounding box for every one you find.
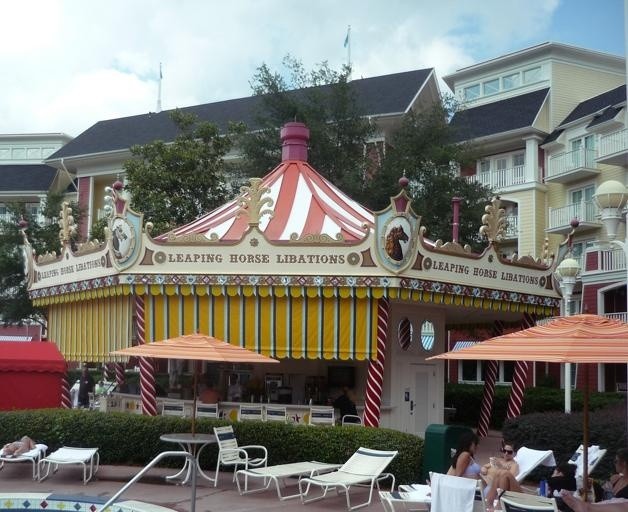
[160,433,218,485]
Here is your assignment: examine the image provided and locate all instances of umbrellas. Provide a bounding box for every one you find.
[110,331,281,439]
[425,311,628,505]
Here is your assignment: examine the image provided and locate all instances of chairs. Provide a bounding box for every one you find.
[0,443,48,479]
[308,407,335,427]
[299,446,398,511]
[162,401,185,417]
[342,414,363,428]
[265,406,286,421]
[236,460,345,500]
[213,424,268,491]
[195,402,224,421]
[38,446,100,486]
[88,393,141,414]
[378,444,607,512]
[240,404,263,422]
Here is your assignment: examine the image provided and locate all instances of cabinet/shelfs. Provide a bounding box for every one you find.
[305,375,328,405]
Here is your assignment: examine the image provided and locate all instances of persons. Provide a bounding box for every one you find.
[329,389,361,427]
[556,488,628,512]
[78,367,95,406]
[445,435,482,480]
[224,373,245,401]
[600,448,628,499]
[485,462,577,512]
[472,439,521,491]
[197,378,224,404]
[1,434,37,460]
[97,381,107,395]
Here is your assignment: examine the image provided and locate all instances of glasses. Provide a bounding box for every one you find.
[503,449,513,454]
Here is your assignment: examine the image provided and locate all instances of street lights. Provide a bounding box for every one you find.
[552,253,582,414]
[591,181,628,267]
